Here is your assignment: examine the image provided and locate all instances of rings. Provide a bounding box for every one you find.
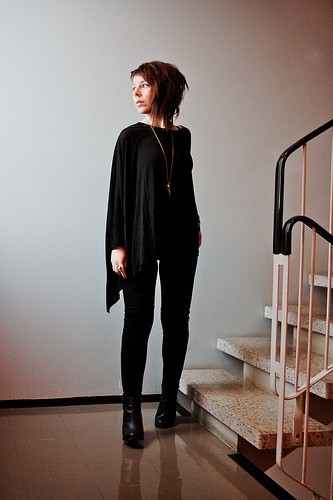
[118,266,122,272]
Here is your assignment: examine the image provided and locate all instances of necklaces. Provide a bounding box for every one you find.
[145,117,178,195]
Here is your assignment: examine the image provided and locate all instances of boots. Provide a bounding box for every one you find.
[122,393,144,441]
[155,384,178,428]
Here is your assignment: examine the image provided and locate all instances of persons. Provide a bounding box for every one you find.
[106,60,202,449]
